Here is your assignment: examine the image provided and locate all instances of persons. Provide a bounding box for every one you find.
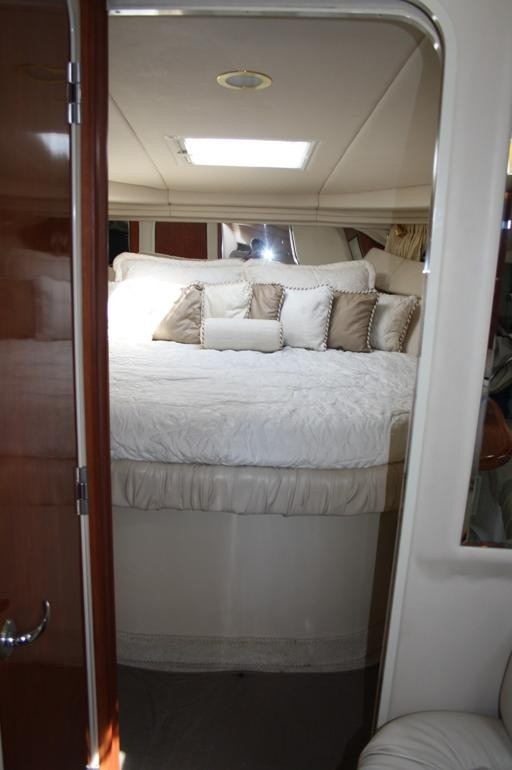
[243,238,269,261]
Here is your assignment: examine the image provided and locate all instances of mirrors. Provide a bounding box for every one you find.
[458,133,512,549]
[98,0,447,769]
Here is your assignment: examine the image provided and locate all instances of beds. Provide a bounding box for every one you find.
[109,250,418,676]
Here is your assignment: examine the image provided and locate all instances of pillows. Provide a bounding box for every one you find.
[244,256,375,291]
[365,248,424,353]
[202,320,284,351]
[370,288,416,353]
[152,284,201,344]
[114,251,245,282]
[282,282,335,351]
[250,282,286,323]
[328,286,379,352]
[200,282,250,319]
